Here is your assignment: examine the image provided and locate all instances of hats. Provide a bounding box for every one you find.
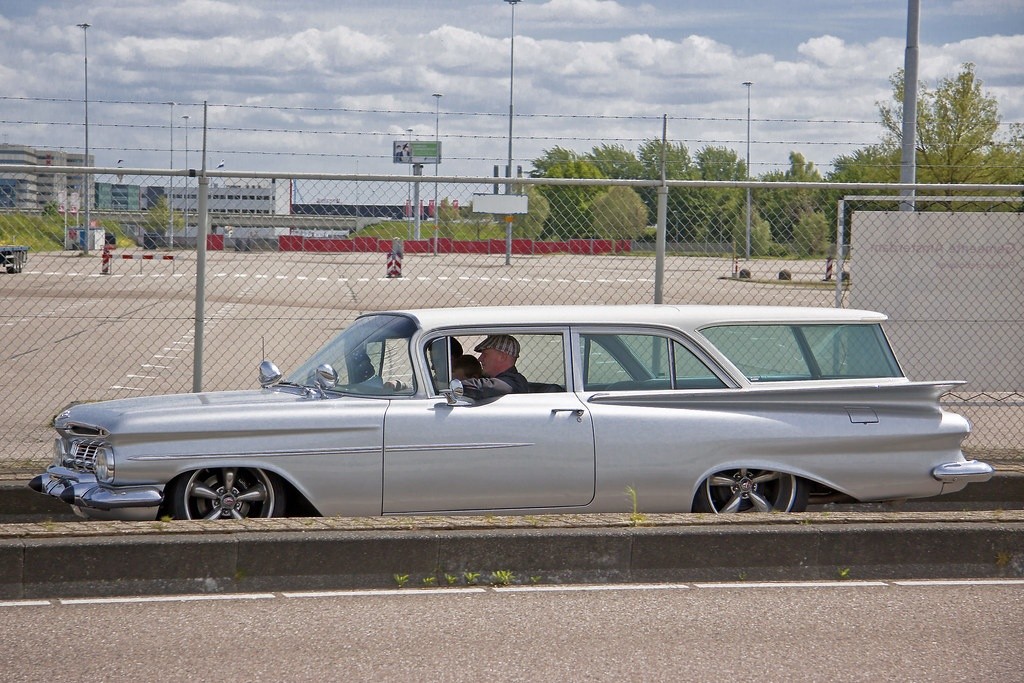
[474,335,520,357]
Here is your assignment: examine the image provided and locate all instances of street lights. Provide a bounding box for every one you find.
[407,127,413,239]
[163,101,175,251]
[76,22,91,255]
[182,115,191,250]
[742,81,754,261]
[505,0,520,263]
[432,92,443,256]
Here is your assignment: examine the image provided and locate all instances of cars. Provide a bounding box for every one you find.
[26,303,996,526]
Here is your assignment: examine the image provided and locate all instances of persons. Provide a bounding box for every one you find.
[424,336,463,379]
[460,334,530,400]
[450,354,482,381]
